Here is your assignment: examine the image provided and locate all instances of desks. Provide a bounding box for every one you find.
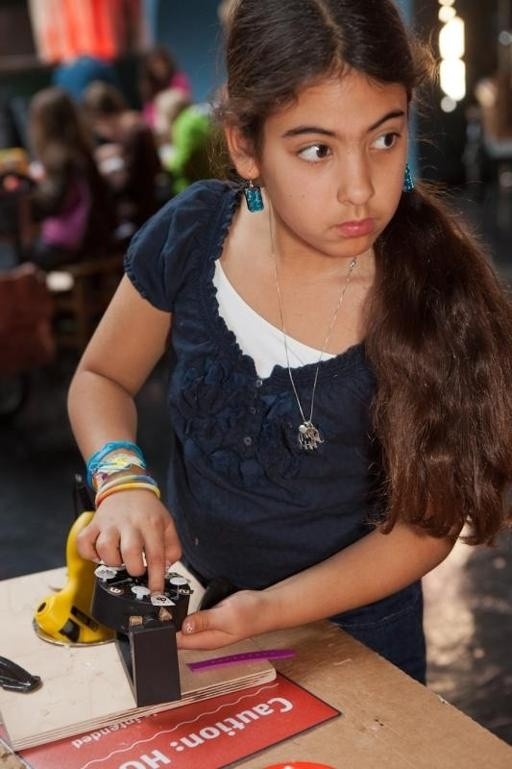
[1,620,510,765]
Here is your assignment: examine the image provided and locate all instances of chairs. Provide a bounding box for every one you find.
[0,173,129,432]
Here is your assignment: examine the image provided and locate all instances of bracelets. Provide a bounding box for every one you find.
[85,442,160,507]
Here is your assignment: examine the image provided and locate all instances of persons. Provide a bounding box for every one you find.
[0,40,232,435]
[68,0,512,686]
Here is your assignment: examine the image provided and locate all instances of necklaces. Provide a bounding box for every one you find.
[268,197,357,450]
